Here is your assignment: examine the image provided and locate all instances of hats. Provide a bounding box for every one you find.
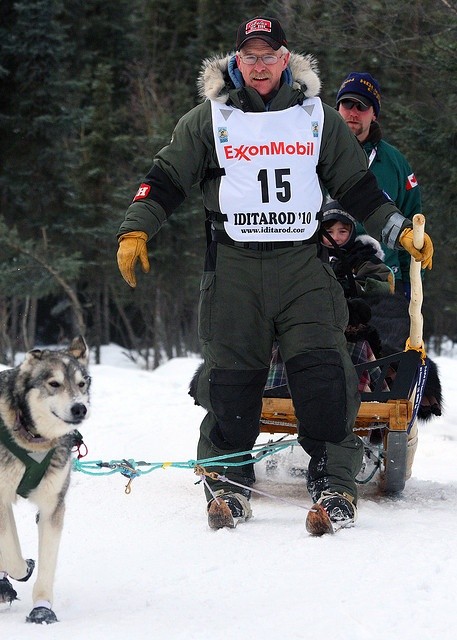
[236,18,287,51]
[337,74,382,115]
[320,203,356,254]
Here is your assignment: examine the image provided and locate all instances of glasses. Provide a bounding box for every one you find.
[239,55,283,66]
[340,99,371,111]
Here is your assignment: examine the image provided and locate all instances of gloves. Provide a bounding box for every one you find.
[399,227,434,270]
[117,231,150,288]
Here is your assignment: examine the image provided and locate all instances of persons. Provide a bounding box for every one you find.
[337,73,423,293]
[117,15,434,522]
[320,201,396,298]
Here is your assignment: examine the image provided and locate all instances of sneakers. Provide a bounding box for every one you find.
[223,491,249,518]
[322,490,355,524]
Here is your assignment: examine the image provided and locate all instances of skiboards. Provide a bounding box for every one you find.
[207,475,357,534]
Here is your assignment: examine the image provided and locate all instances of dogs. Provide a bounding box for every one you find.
[0,334,90,625]
